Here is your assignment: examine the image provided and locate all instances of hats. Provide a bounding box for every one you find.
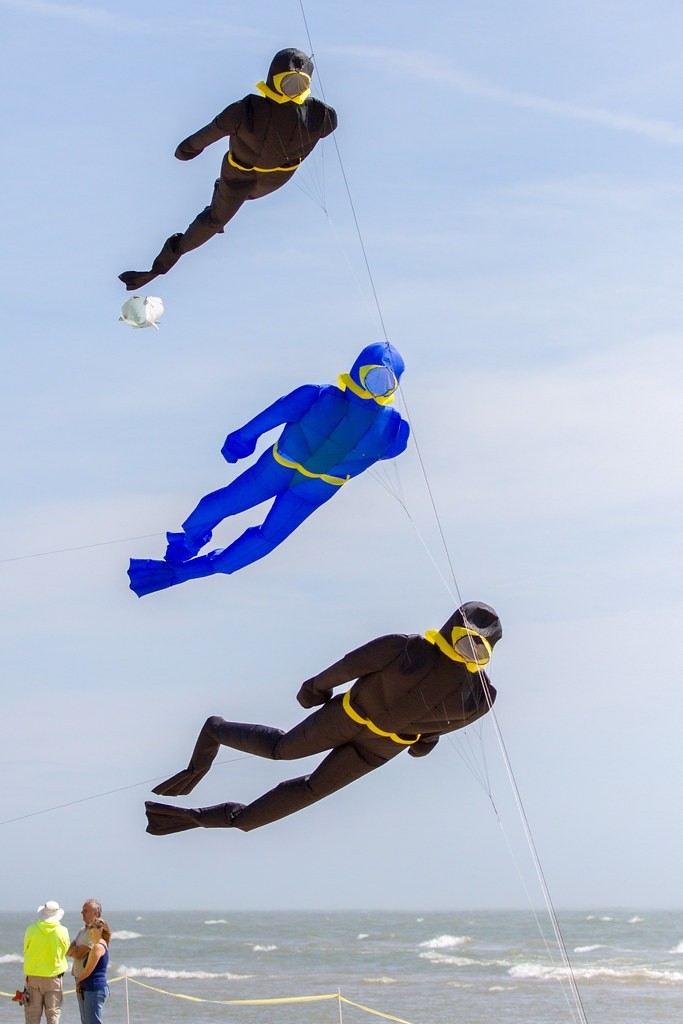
[37,901,64,923]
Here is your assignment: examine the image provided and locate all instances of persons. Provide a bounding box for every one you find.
[66,899,102,1024]
[76,918,111,1024]
[23,901,71,1024]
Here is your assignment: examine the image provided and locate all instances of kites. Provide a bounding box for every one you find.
[145,602,502,836]
[125,342,410,599]
[120,48,340,292]
